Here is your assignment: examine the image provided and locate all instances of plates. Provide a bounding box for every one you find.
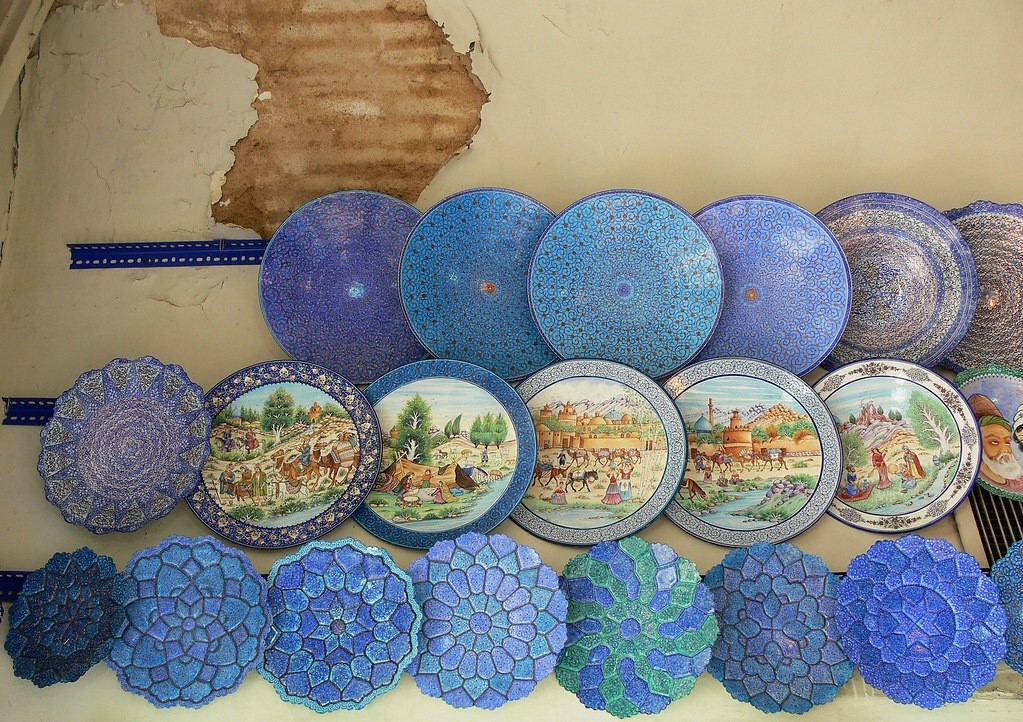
[4,187,1023,719]
